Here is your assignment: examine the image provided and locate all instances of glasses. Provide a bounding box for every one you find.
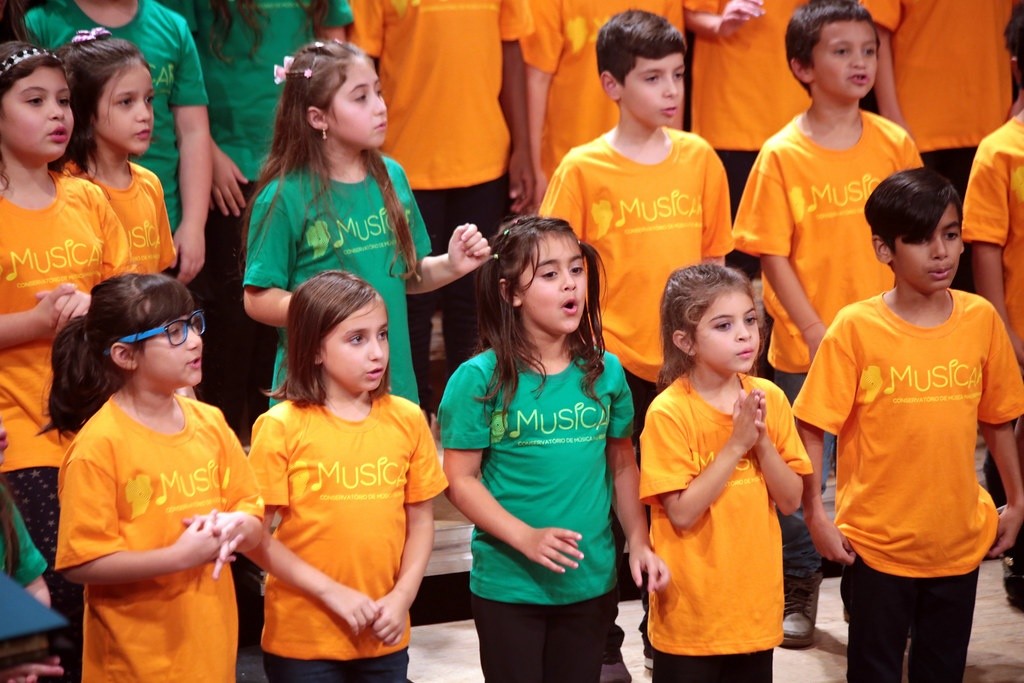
[103,308,206,356]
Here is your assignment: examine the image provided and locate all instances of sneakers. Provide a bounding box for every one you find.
[779,571,823,647]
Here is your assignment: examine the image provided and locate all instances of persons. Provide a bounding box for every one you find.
[734,0,923,651]
[242,40,489,413]
[639,264,815,683]
[48,28,177,275]
[0,419,65,683]
[0,37,135,603]
[241,269,449,683]
[962,0,1024,613]
[55,273,265,683]
[0,0,1024,436]
[791,167,1024,683]
[539,8,735,463]
[437,217,668,683]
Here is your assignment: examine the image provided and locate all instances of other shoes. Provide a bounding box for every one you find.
[599,647,632,683]
[1003,555,1024,612]
[642,634,653,670]
